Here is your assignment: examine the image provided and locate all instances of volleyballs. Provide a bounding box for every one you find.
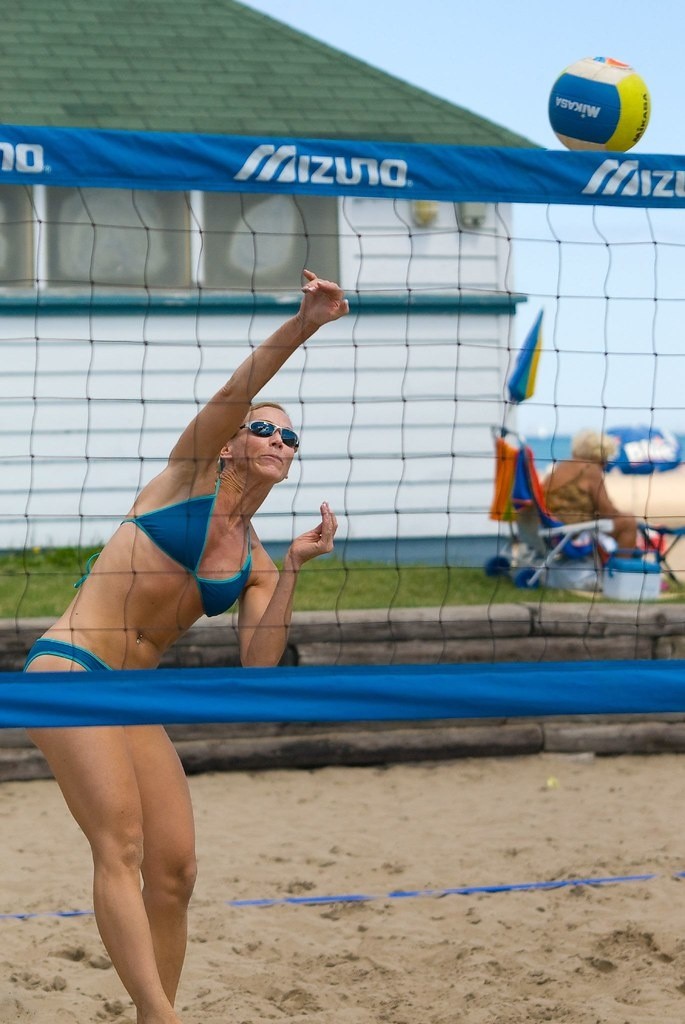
[545,55,653,152]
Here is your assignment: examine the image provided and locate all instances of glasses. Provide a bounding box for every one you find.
[241,420,299,449]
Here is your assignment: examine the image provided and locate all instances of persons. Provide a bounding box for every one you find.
[17,268,351,1024]
[542,437,637,559]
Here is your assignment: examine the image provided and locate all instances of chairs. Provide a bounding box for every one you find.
[487,424,685,592]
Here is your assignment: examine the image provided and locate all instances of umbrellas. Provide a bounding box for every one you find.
[501,304,546,428]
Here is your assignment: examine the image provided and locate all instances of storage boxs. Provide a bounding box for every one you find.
[603,547,662,602]
